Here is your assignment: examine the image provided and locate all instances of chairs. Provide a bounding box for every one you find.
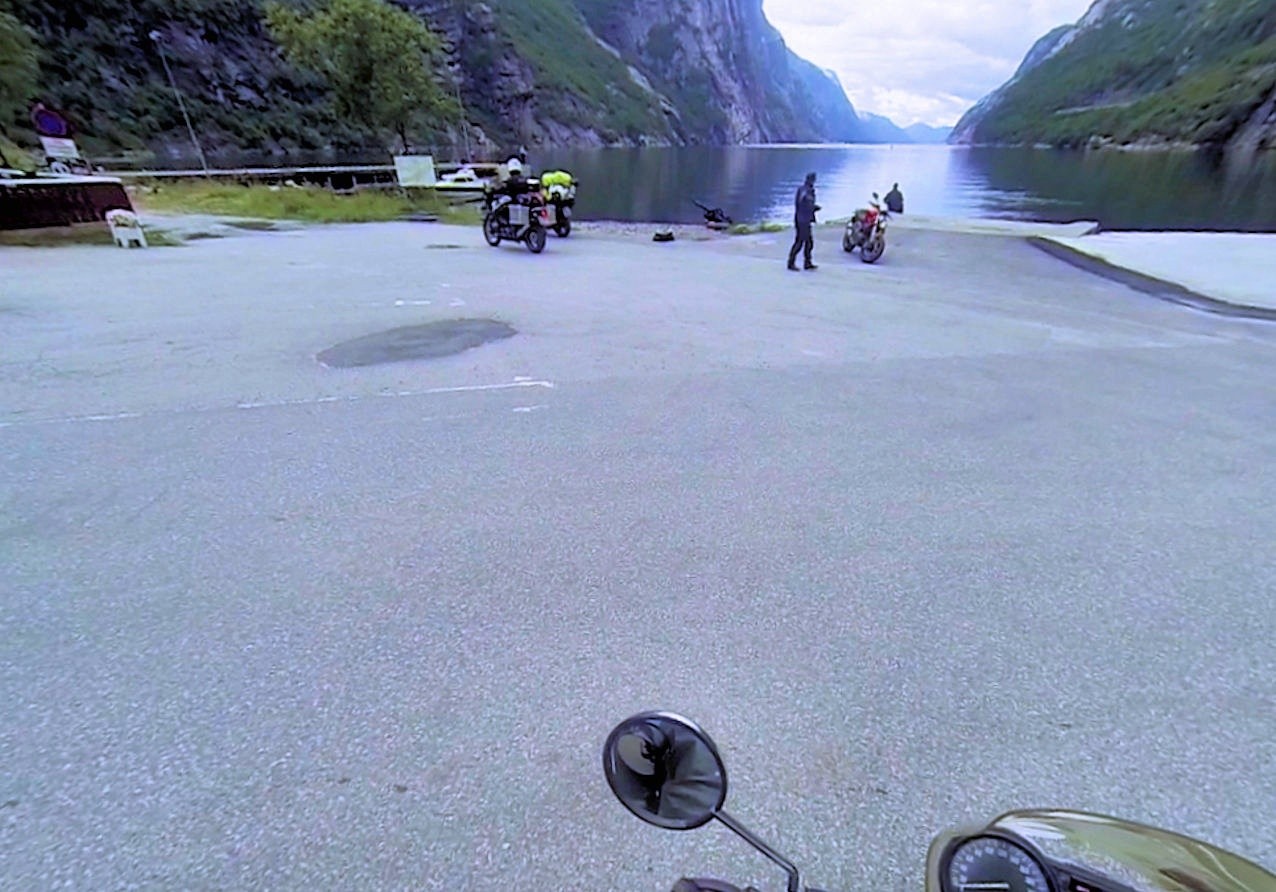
[108,209,147,248]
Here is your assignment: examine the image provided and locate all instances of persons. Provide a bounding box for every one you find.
[507,143,528,178]
[788,172,821,270]
[461,150,476,165]
[884,183,903,213]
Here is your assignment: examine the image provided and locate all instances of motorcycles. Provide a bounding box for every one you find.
[602,707,1276,892]
[843,191,891,263]
[481,178,577,254]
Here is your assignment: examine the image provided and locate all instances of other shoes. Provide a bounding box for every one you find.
[804,264,818,270]
[787,264,800,271]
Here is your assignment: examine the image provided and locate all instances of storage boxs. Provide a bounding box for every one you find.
[509,204,530,225]
[539,204,556,225]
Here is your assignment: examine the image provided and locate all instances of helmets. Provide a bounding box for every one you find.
[507,156,522,177]
